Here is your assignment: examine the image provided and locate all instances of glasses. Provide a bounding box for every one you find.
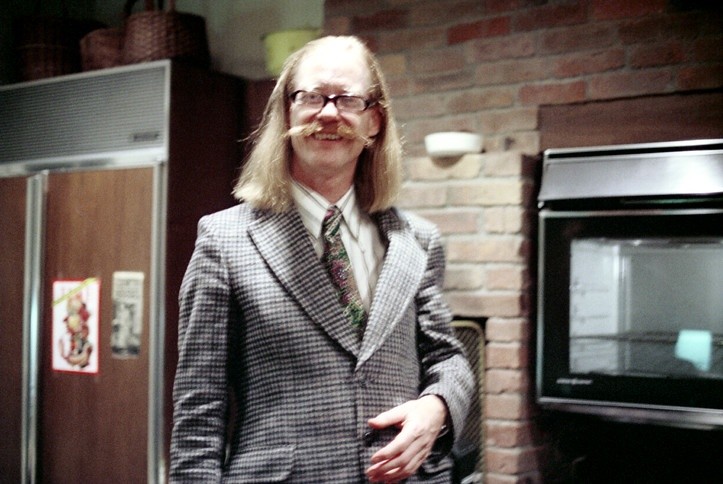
[288,90,383,112]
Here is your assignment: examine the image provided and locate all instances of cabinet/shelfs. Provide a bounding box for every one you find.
[0,57,244,484]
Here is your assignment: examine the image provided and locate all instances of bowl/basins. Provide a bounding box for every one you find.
[260,30,319,78]
[425,131,483,157]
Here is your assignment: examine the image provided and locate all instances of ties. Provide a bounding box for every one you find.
[320,206,369,345]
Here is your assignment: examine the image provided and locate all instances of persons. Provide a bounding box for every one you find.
[164,35,475,484]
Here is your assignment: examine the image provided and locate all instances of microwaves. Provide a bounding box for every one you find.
[536,140,723,432]
